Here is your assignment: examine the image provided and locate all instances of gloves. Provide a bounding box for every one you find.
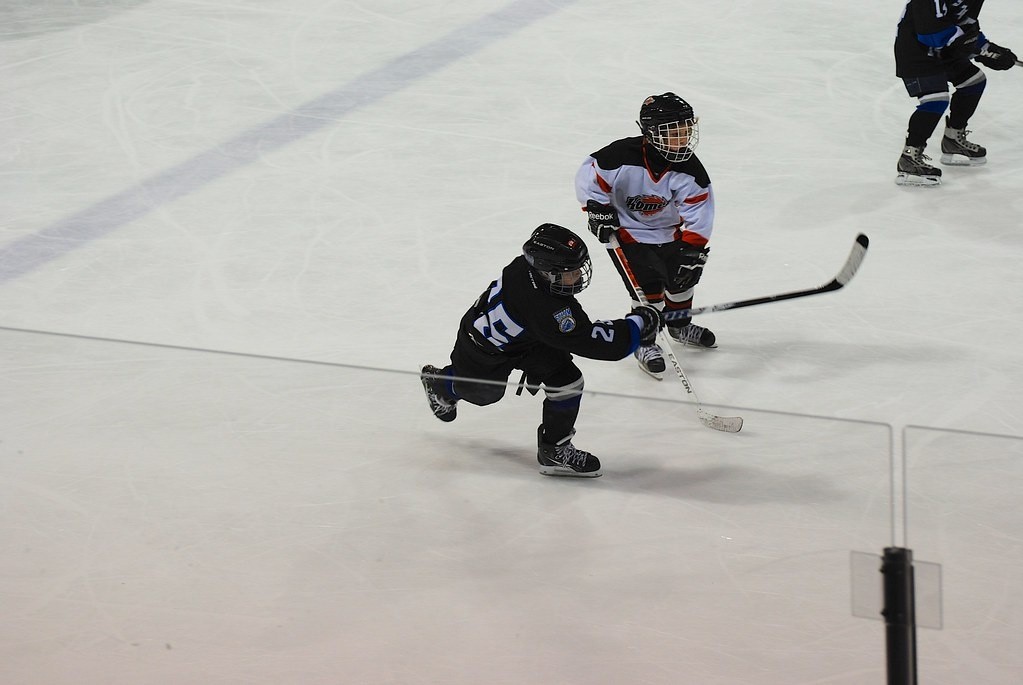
[586,200,619,243]
[929,46,948,79]
[673,243,710,290]
[625,304,664,339]
[975,43,1018,70]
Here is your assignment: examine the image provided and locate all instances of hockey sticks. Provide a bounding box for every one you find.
[608,233,744,434]
[656,232,871,323]
[975,47,1023,68]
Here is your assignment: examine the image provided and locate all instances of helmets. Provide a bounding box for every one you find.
[522,223,592,297]
[640,92,699,164]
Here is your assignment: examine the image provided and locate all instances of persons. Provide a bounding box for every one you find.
[574,92,718,381]
[420,223,664,478]
[893,0,1018,186]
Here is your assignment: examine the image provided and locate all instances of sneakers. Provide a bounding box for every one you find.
[895,138,942,187]
[667,323,719,349]
[941,116,986,166]
[634,346,666,380]
[421,364,460,422]
[537,425,601,477]
